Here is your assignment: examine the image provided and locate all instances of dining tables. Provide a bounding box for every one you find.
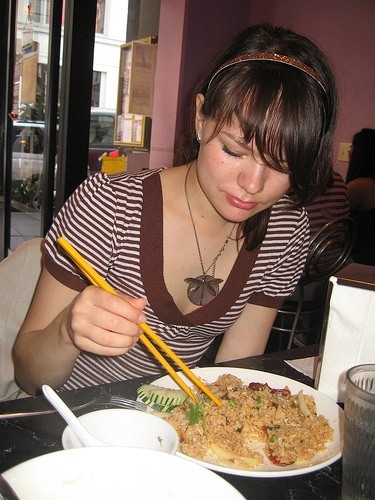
[0,344,375,500]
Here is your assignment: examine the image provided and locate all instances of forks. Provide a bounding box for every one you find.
[0,395,147,419]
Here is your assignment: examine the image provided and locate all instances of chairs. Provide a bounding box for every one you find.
[269,216,358,350]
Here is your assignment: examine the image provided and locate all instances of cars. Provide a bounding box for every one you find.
[88,106,118,166]
[8,120,59,209]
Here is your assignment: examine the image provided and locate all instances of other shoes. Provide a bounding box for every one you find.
[294,333,313,347]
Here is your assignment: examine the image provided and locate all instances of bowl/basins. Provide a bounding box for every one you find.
[0,446,247,500]
[61,409,179,454]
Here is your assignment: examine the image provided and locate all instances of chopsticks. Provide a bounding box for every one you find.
[55,235,220,409]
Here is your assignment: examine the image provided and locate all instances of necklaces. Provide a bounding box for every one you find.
[184,159,238,306]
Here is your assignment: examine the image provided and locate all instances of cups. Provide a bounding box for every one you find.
[342,364,375,500]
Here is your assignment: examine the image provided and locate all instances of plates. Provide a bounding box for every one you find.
[135,367,342,478]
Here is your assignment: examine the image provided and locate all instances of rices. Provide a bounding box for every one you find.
[166,373,334,468]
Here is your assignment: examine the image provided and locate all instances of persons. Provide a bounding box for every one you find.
[10,24,339,396]
[285,128,375,348]
